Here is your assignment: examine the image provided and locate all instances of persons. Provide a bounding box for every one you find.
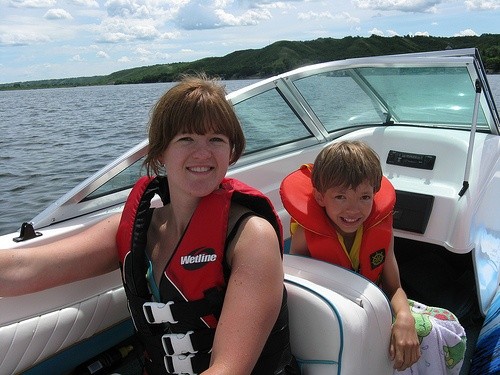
[279,140,468,375]
[0,72,303,375]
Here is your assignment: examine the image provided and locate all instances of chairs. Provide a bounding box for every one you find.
[282,254,393,375]
